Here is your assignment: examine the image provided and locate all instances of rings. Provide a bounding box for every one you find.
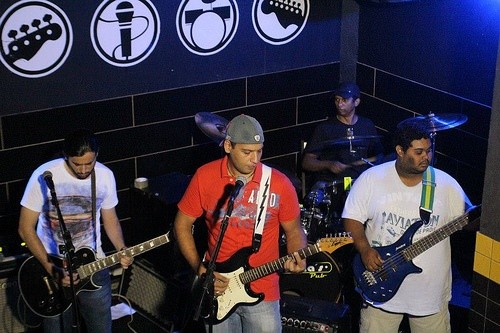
[216,290,219,295]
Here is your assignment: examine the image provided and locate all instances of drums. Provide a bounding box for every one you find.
[300,180,355,232]
[278,235,351,304]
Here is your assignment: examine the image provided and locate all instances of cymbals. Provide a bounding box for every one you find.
[195,112,230,143]
[317,135,386,142]
[396,113,469,131]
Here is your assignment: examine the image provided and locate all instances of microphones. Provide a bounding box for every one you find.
[42,171,55,192]
[231,176,248,203]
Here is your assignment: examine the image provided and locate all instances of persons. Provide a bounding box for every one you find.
[18,129,134,333]
[343,123,480,333]
[174,115,307,333]
[302,85,382,175]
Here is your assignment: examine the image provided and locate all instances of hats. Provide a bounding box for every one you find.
[329,82,359,99]
[219,114,264,148]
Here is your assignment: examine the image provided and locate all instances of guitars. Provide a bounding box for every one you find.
[352,202,484,303]
[193,232,355,324]
[18,231,173,318]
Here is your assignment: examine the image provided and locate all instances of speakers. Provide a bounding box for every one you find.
[0,249,187,333]
